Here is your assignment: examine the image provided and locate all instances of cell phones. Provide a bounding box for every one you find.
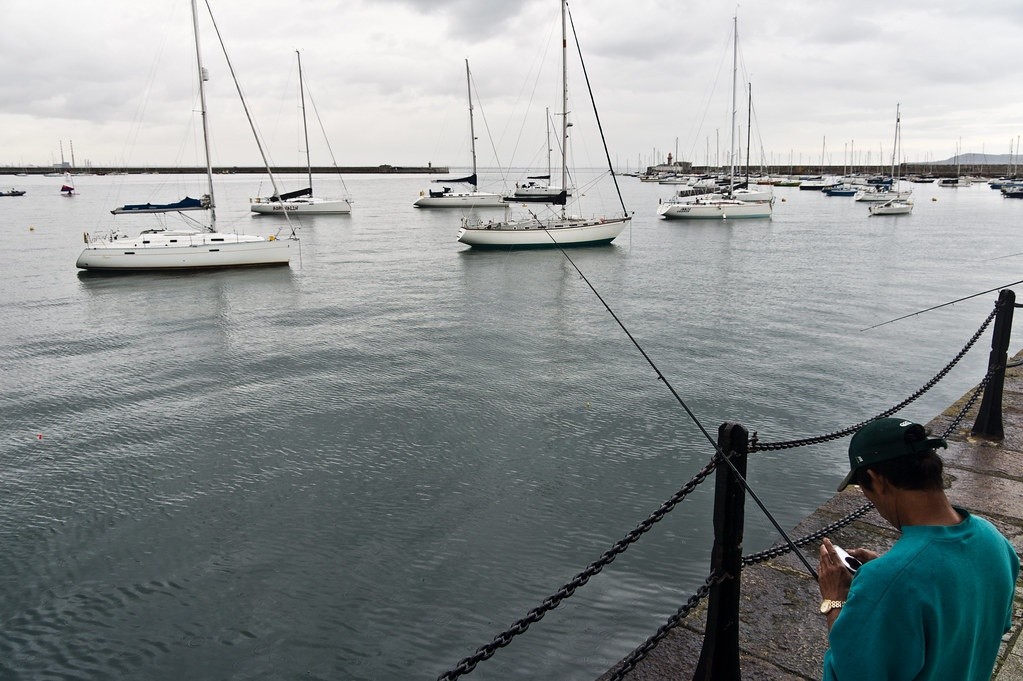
[832,544,862,573]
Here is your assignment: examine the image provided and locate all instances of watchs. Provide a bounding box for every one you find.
[819,598,846,614]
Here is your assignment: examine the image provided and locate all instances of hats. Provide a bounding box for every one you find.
[837,418,948,492]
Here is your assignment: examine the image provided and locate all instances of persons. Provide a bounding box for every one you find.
[818,416,1021,681]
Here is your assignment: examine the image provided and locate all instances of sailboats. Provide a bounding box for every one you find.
[774,103,913,216]
[76,0,301,266]
[610,15,786,219]
[251,48,352,215]
[457,0,633,249]
[911,135,1023,198]
[412,58,509,208]
[514,108,575,196]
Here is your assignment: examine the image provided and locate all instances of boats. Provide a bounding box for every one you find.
[0,191,26,196]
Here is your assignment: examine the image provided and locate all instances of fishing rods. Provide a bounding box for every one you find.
[530,208,818,584]
[860,281,1023,331]
